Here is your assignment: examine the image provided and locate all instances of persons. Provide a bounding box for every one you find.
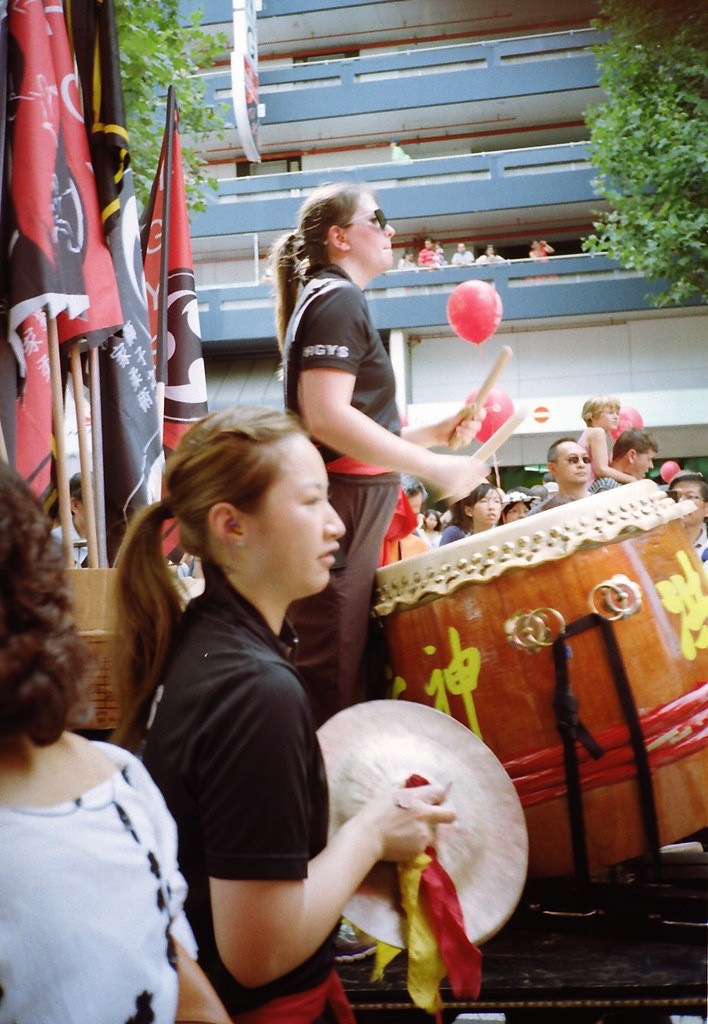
[106,403,457,1024]
[668,473,708,561]
[588,428,659,494]
[451,242,474,264]
[270,182,492,730]
[476,244,505,263]
[388,473,559,566]
[528,241,555,262]
[399,237,448,269]
[0,460,235,1024]
[578,395,637,491]
[528,438,591,516]
[51,472,89,567]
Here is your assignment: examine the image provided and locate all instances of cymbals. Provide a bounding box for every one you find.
[312,695,534,954]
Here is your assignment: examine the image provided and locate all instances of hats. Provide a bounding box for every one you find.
[501,491,540,508]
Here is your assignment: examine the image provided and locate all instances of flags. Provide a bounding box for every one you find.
[0,0,209,565]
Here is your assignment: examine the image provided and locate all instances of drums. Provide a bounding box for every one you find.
[369,474,708,885]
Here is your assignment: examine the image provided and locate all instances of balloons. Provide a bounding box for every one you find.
[660,461,681,483]
[466,387,514,443]
[610,408,643,441]
[447,280,502,344]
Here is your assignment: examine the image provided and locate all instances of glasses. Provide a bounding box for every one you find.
[558,456,590,466]
[342,209,386,232]
[677,496,705,503]
